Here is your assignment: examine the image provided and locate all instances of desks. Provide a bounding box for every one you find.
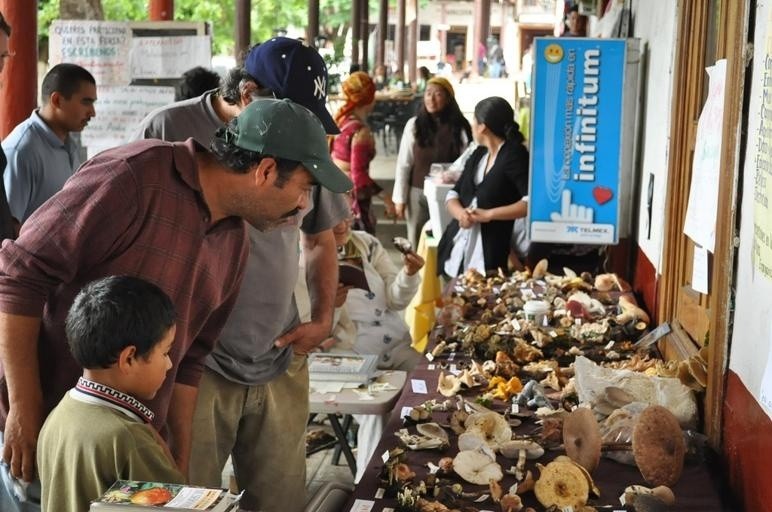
[344,276,725,512]
[305,367,409,479]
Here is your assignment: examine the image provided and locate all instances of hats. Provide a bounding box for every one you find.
[246,35,341,134]
[343,72,376,106]
[228,97,354,193]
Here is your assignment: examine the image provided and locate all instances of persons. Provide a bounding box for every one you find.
[173,66,222,106]
[435,94,529,285]
[323,72,393,238]
[35,274,185,511]
[293,188,425,485]
[391,76,474,251]
[0,15,23,249]
[1,63,97,235]
[124,36,354,510]
[0,95,354,511]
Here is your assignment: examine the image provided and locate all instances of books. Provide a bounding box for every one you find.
[306,350,380,384]
[88,478,246,511]
